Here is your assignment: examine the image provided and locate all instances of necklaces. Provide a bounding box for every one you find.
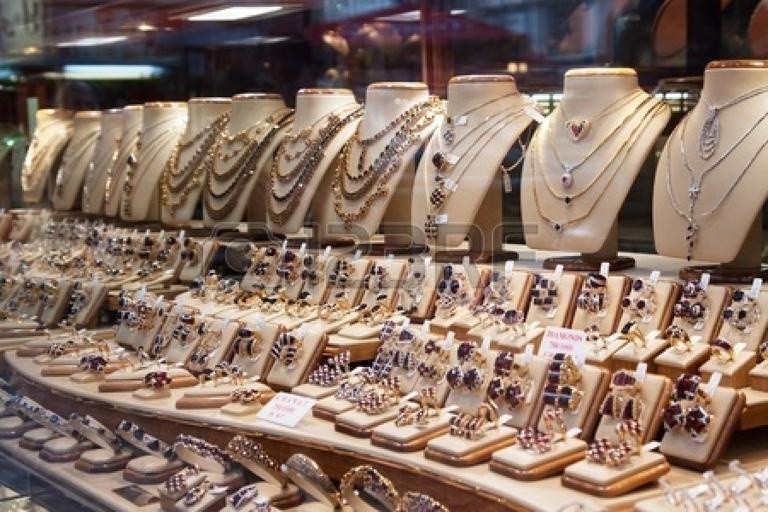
[18,86,768,264]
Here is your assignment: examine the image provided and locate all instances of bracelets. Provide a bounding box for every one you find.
[0,388,448,512]
[656,459,768,510]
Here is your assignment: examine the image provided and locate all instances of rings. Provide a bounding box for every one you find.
[1,221,768,471]
